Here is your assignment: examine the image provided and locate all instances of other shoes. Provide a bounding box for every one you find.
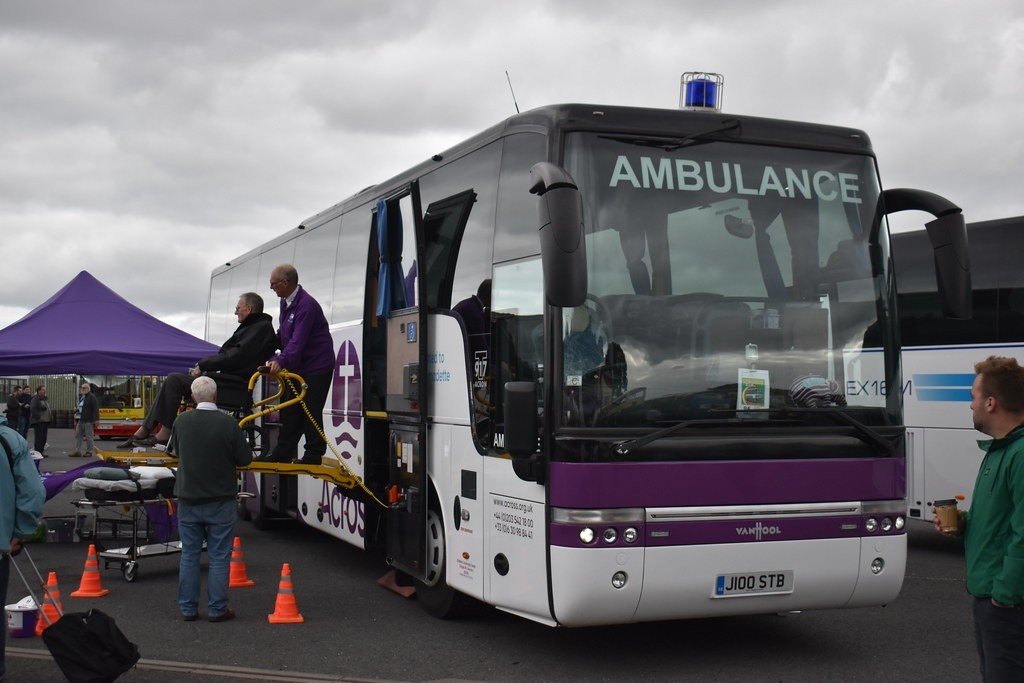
[131,436,169,447]
[301,451,321,465]
[116,434,151,448]
[83,451,92,457]
[68,450,82,457]
[42,453,49,457]
[208,607,234,622]
[183,612,198,621]
[256,454,292,463]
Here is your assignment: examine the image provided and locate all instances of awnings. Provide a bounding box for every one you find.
[0,270,222,373]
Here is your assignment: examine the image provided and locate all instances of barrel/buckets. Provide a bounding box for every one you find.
[4,604,41,638]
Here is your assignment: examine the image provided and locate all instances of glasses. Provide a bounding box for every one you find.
[235,307,240,311]
[270,280,277,286]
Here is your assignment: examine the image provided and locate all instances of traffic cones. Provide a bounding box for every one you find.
[68,544,109,598]
[34,570,65,636]
[267,562,304,624]
[228,535,254,587]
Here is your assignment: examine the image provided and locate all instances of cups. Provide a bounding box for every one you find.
[933,499,958,533]
[745,344,758,361]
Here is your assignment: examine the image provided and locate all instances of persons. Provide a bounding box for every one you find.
[268,264,335,464]
[166,376,252,622]
[931,356,1024,683]
[0,384,100,683]
[116,292,276,449]
[451,279,534,444]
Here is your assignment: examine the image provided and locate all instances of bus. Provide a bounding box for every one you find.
[891,216,1024,524]
[204,71,973,628]
[0,375,30,421]
[73,374,194,441]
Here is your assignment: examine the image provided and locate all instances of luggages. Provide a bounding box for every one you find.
[6,543,141,683]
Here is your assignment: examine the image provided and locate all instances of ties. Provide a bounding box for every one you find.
[281,300,287,311]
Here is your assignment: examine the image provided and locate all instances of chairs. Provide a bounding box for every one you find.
[597,291,754,359]
